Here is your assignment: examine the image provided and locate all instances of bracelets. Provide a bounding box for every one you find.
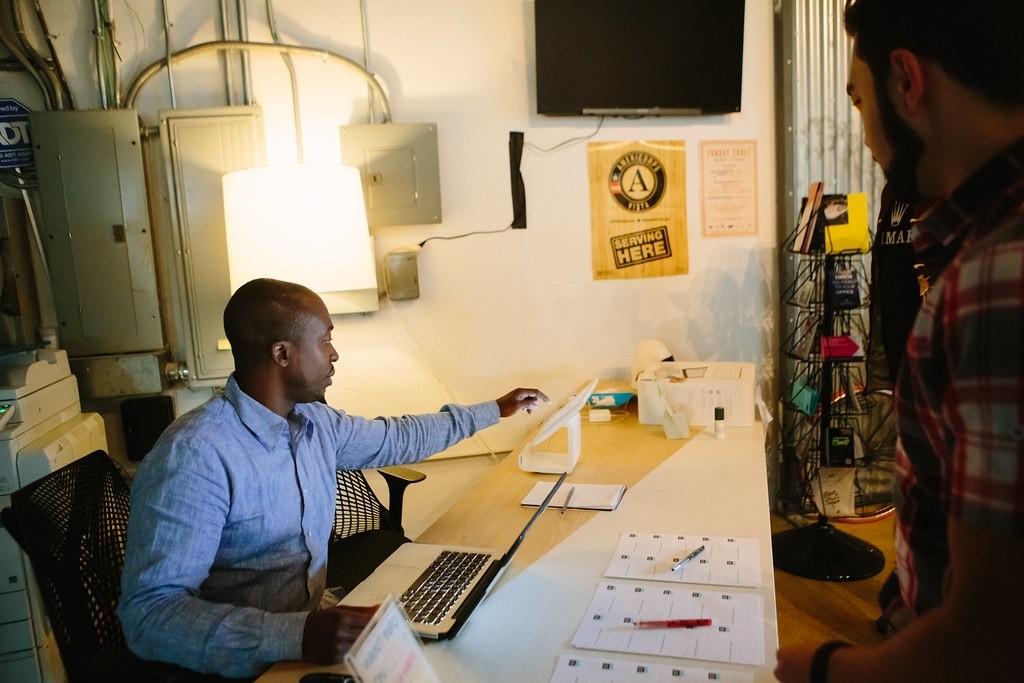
[810,641,851,683]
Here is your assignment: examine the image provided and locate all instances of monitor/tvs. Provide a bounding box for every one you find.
[535,0,745,117]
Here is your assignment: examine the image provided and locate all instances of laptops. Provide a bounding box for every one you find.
[336,472,568,641]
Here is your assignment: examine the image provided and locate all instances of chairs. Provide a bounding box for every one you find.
[0,450,208,683]
[319,396,427,604]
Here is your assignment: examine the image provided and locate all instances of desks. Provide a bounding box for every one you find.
[252,379,780,683]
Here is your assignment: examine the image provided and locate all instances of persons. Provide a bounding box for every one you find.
[777,1,1024,683]
[112,277,552,677]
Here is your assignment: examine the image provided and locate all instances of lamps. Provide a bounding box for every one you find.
[221,159,379,316]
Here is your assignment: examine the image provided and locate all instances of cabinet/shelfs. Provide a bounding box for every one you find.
[780,206,877,518]
[157,105,264,389]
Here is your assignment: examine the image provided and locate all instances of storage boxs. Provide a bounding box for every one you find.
[638,361,755,427]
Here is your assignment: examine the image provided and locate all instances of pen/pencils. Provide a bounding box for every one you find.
[561,487,576,515]
[634,618,713,630]
[671,546,705,571]
